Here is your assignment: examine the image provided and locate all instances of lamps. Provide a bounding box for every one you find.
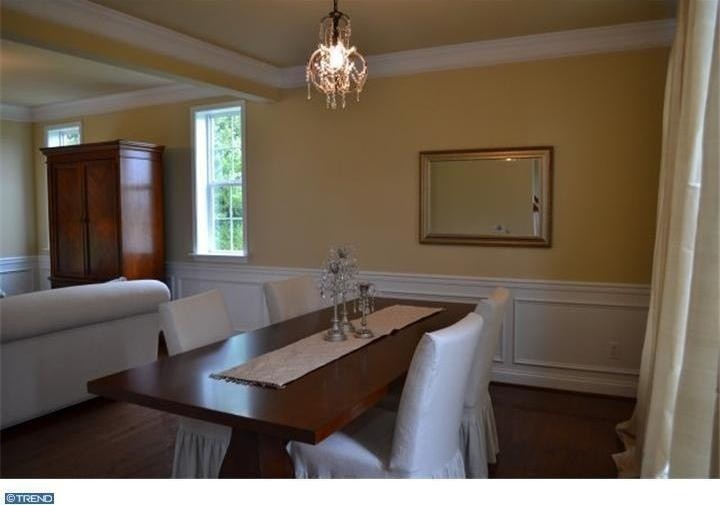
[303,1,368,111]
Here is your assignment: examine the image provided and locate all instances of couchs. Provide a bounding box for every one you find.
[0,278,172,432]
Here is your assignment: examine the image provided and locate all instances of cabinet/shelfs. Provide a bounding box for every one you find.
[38,138,167,354]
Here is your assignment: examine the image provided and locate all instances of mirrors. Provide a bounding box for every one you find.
[416,144,554,248]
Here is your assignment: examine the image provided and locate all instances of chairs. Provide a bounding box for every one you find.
[380,287,510,479]
[154,289,232,479]
[262,272,326,325]
[286,310,484,479]
